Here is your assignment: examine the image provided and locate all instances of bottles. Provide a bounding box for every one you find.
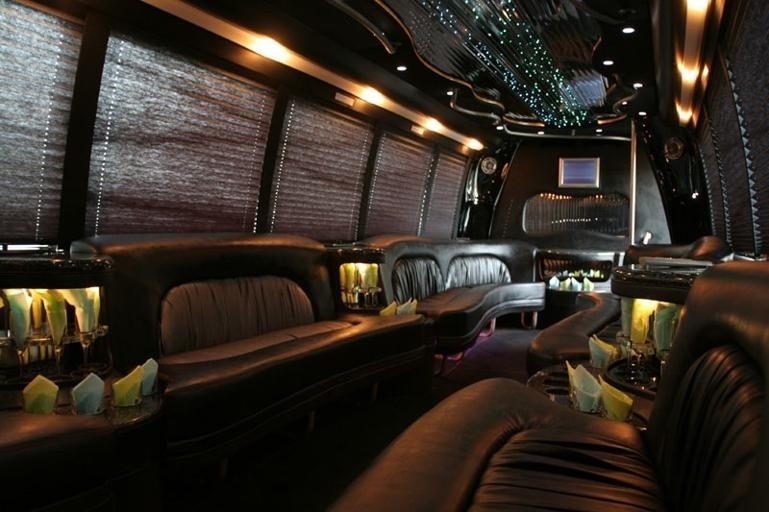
[1,327,113,386]
[623,336,670,388]
[347,286,381,308]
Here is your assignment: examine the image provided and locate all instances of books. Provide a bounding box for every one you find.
[639,256,713,274]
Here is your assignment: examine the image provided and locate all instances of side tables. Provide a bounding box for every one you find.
[527,358,656,427]
[0,366,163,512]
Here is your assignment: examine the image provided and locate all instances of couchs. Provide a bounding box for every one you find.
[68,234,439,511]
[527,235,731,376]
[360,235,547,373]
[318,261,769,511]
